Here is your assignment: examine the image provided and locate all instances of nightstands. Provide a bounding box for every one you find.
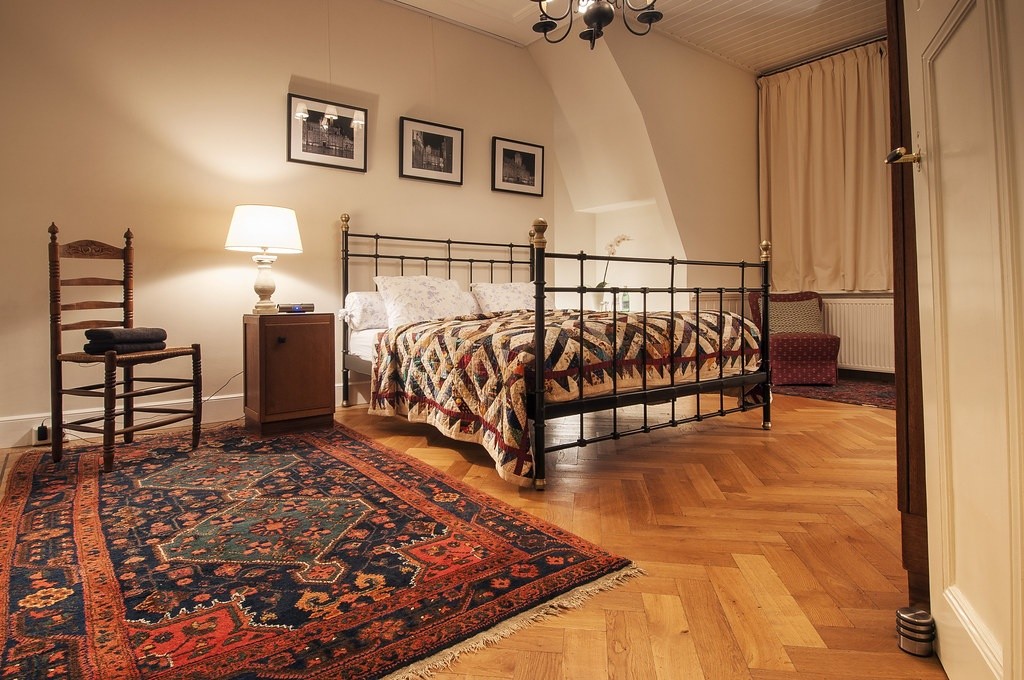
[243,313,336,433]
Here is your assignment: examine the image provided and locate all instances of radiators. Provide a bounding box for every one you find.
[821,298,895,372]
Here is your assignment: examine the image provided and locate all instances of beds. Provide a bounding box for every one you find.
[339,213,772,491]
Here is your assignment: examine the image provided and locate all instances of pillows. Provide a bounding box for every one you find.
[469,281,552,314]
[339,290,482,331]
[374,276,471,328]
[758,298,824,335]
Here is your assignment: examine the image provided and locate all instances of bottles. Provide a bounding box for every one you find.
[621,286,629,311]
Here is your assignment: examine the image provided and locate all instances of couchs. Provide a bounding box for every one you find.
[748,292,840,386]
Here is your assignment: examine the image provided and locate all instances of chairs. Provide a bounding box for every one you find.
[48,221,202,472]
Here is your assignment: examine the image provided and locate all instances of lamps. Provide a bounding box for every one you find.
[224,204,304,315]
[531,0,663,51]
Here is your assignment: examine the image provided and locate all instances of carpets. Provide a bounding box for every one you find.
[771,376,897,411]
[0,420,647,680]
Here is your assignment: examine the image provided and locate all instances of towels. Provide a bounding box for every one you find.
[83,341,167,354]
[85,327,168,342]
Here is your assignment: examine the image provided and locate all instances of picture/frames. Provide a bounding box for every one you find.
[399,117,464,185]
[492,136,545,196]
[286,92,368,173]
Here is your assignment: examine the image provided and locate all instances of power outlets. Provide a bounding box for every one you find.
[32,426,52,445]
